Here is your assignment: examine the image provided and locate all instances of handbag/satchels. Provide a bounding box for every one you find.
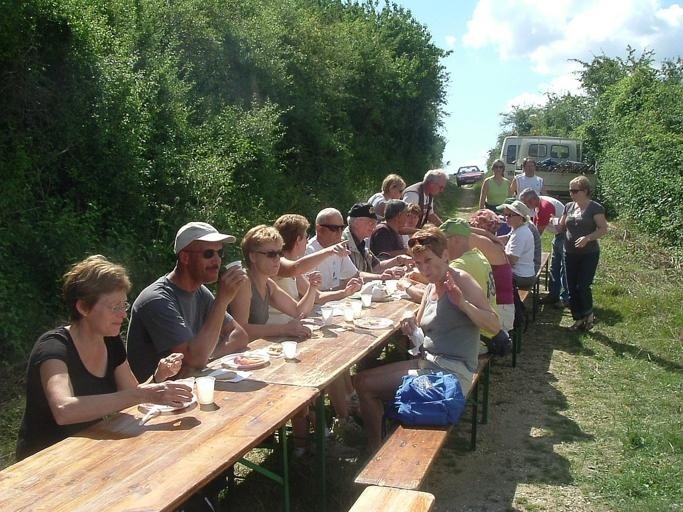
[383,372,466,426]
[480,329,513,354]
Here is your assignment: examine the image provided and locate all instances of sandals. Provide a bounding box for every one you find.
[567,316,594,332]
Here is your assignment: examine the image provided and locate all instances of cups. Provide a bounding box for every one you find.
[175,379,194,392]
[225,261,242,271]
[343,293,372,322]
[281,341,297,359]
[320,306,333,326]
[196,376,216,405]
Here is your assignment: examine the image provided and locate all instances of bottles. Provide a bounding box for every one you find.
[372,281,386,302]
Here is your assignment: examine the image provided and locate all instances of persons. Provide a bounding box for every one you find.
[125,222,250,383]
[14,253,194,462]
[548,175,607,330]
[478,157,568,306]
[225,168,518,463]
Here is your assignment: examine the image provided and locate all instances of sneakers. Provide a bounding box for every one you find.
[539,293,569,307]
[289,403,363,485]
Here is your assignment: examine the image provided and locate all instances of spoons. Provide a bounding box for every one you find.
[139,405,166,426]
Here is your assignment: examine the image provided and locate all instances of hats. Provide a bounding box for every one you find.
[438,217,471,238]
[348,202,377,219]
[173,222,236,254]
[468,208,501,231]
[502,200,531,221]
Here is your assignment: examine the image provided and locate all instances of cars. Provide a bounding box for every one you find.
[454,166,485,186]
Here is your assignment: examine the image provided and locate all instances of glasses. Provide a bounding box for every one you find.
[254,249,284,257]
[408,236,434,248]
[105,302,130,313]
[204,249,224,258]
[320,224,345,232]
[504,213,517,217]
[494,165,502,168]
[569,190,584,194]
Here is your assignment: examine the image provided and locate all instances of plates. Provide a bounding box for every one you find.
[354,316,393,329]
[221,351,270,369]
[140,392,197,412]
[301,318,326,329]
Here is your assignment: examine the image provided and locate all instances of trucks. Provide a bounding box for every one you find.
[501,135,596,203]
